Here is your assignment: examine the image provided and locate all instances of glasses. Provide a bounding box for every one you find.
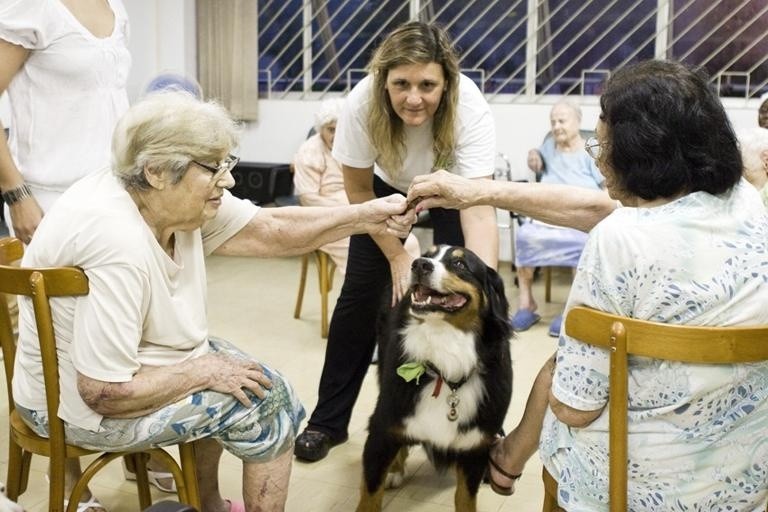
[585,136,612,161]
[193,153,240,181]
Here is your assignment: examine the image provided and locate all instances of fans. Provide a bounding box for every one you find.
[140,73,204,106]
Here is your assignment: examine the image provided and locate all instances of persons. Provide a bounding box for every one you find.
[293,98,420,371]
[744,150,766,205]
[11,88,418,512]
[508,100,608,336]
[294,23,497,461]
[1,0,178,512]
[405,59,768,512]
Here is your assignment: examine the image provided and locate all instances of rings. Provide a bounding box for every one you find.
[27,235,31,239]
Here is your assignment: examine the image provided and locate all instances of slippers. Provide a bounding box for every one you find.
[508,310,540,333]
[549,315,563,337]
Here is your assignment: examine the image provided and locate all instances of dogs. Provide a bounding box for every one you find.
[356,244,520,512]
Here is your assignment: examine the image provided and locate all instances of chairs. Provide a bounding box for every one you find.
[291,150,338,337]
[533,302,764,507]
[532,129,612,302]
[1,236,235,509]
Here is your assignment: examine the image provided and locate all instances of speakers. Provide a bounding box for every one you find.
[227,163,296,206]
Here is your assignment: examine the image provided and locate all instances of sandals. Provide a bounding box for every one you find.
[45,472,108,512]
[123,460,177,492]
[487,429,521,495]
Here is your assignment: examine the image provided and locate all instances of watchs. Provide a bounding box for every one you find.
[2,185,31,205]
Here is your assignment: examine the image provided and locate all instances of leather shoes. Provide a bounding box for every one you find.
[296,427,348,460]
